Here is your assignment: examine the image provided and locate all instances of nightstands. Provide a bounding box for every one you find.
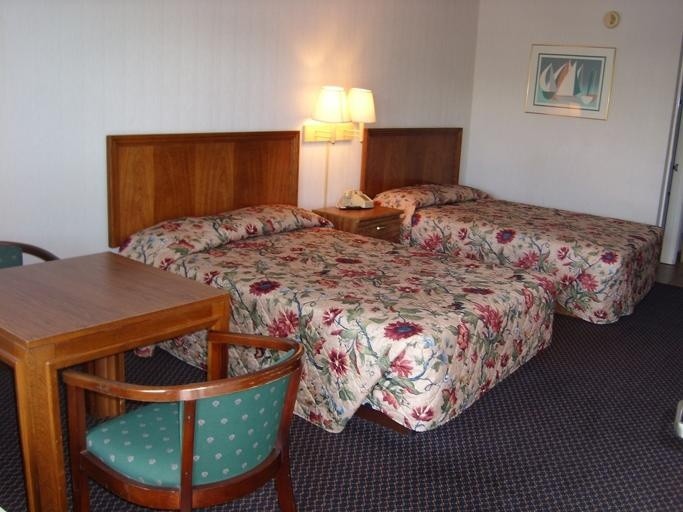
[312,205,404,244]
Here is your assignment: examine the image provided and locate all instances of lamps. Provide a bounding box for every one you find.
[303,86,376,145]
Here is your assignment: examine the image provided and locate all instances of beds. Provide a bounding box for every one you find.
[364,126,663,324]
[106,131,554,436]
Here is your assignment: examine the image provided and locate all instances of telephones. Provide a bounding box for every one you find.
[341,190,374,208]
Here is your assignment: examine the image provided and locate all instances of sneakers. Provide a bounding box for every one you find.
[675,400,683,436]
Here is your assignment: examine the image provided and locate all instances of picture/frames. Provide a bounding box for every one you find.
[523,43,617,121]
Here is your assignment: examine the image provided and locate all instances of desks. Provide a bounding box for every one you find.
[1,252,232,510]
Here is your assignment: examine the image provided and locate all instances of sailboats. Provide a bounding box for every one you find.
[540,60,596,102]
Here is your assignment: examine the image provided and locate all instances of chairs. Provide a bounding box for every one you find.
[62,329,305,512]
[1,240,60,269]
